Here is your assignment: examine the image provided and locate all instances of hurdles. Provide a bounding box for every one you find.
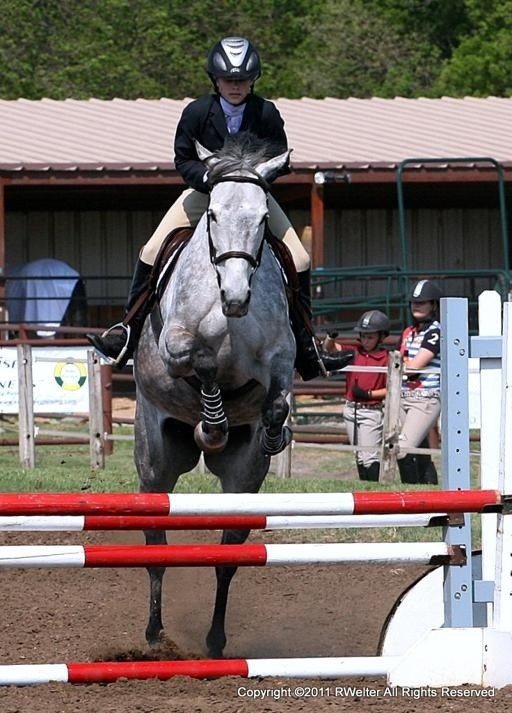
[0,290,511,689]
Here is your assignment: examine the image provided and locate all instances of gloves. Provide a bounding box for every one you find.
[324,327,338,338]
[352,387,371,402]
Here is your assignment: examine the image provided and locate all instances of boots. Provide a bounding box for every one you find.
[87,245,154,371]
[357,461,379,480]
[288,268,353,381]
[399,454,439,485]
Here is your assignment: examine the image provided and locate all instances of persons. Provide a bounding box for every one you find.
[392,277,443,483]
[322,308,392,481]
[86,37,356,380]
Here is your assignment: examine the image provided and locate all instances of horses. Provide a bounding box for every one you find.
[132,133,297,682]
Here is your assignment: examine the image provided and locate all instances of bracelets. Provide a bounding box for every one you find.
[368,388,373,401]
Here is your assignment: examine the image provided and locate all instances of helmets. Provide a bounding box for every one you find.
[206,37,261,81]
[405,279,441,302]
[356,311,393,336]
